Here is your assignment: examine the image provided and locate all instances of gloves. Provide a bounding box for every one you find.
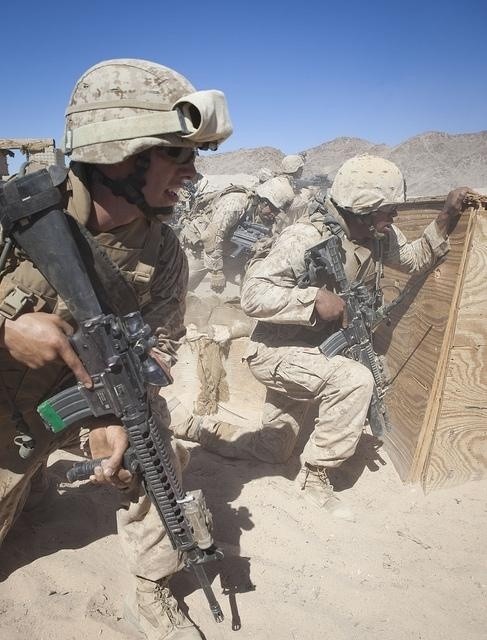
[211,270,226,290]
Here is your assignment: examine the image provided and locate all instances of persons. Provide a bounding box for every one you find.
[174,174,294,297]
[257,155,321,209]
[160,169,212,226]
[160,151,484,516]
[1,56,238,563]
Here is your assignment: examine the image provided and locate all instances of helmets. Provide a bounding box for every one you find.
[61,59,196,163]
[258,154,303,213]
[330,156,404,213]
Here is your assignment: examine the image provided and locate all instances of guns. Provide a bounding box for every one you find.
[227,221,270,258]
[297,236,391,437]
[1,167,225,623]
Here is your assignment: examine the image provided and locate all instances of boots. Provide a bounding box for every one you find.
[123,576,204,639]
[293,462,355,523]
[166,398,201,441]
